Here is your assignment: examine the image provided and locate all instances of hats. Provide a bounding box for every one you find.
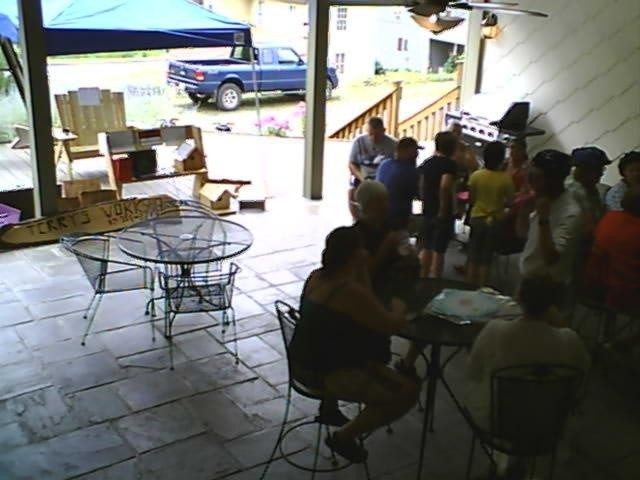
[396,136,424,150]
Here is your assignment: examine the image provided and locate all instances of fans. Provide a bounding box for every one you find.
[328,0,549,17]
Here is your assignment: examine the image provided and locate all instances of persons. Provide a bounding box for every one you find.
[348,117,638,380]
[287,227,419,465]
[473,267,590,478]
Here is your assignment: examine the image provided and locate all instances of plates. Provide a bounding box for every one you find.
[432,293,500,317]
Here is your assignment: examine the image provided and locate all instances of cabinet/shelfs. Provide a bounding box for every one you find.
[96,124,209,201]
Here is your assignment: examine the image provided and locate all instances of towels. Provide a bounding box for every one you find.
[79,87,101,105]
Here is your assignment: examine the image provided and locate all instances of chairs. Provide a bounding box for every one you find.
[54,89,126,170]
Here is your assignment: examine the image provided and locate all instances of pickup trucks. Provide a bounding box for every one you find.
[161,40,341,111]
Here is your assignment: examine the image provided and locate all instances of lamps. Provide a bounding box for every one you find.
[480,14,498,39]
[409,14,465,34]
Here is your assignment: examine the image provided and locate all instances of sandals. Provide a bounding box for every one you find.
[323,435,369,464]
[314,408,350,428]
